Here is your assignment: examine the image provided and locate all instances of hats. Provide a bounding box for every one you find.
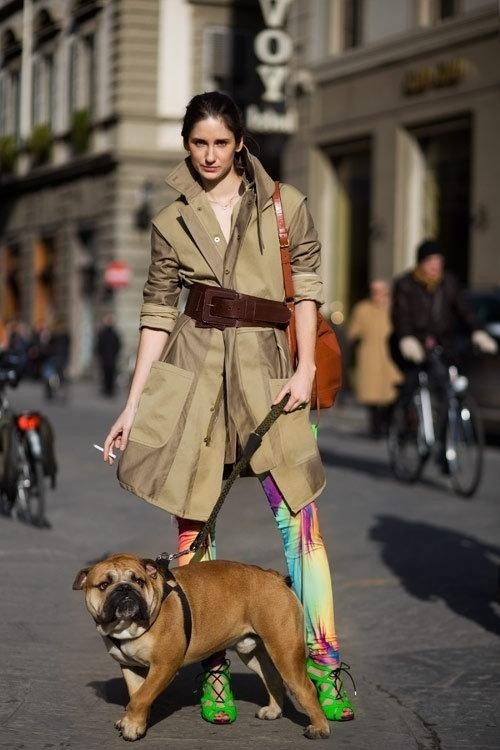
[417,241,447,265]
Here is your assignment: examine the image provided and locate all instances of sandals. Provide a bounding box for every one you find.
[304,656,355,722]
[198,657,237,726]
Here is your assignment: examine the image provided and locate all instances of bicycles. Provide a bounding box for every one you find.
[385,344,490,501]
[0,366,54,530]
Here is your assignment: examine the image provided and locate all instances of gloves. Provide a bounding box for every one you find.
[399,335,426,363]
[470,329,498,355]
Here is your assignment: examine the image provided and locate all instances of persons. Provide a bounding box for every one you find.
[100,89,360,727]
[1,309,124,406]
[385,234,496,478]
[342,272,407,436]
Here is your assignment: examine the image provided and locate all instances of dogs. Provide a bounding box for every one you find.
[71,551,331,742]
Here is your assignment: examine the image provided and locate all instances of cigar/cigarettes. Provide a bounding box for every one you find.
[92,442,117,460]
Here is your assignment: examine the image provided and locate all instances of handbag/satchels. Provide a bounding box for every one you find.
[272,181,344,411]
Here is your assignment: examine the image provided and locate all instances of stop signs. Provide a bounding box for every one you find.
[103,259,134,292]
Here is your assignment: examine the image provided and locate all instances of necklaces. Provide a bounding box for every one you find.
[208,193,242,212]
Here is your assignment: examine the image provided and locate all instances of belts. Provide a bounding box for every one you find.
[183,281,293,332]
